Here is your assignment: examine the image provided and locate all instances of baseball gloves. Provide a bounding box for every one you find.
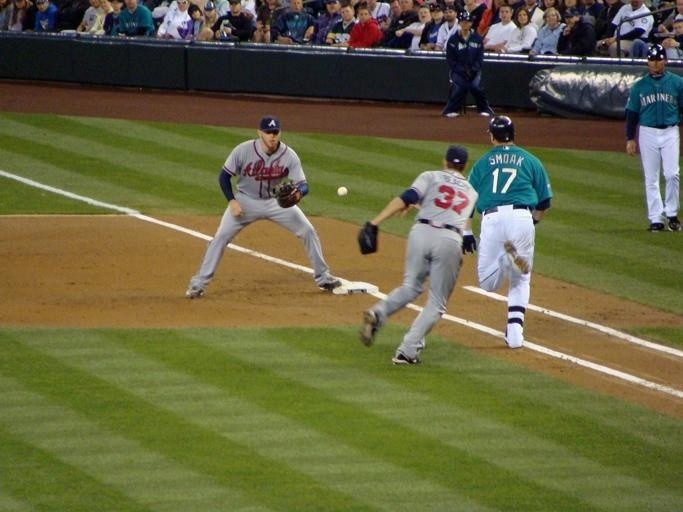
[277,185,303,207]
[357,220,377,254]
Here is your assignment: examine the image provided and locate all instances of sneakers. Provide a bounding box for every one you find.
[318,277,341,290]
[504,326,524,348]
[651,223,663,233]
[186,286,205,298]
[481,112,490,117]
[442,112,458,118]
[392,352,422,365]
[360,308,379,346]
[503,240,528,274]
[667,216,681,232]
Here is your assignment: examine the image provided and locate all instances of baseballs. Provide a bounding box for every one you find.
[337,186,350,198]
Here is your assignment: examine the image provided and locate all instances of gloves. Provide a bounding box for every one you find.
[463,235,476,254]
[358,222,376,253]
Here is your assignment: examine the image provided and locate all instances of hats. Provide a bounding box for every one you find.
[262,117,280,131]
[446,146,466,164]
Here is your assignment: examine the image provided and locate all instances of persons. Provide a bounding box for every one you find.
[462,115,553,347]
[624,44,682,232]
[358,146,479,364]
[0,1,683,60]
[186,117,341,300]
[442,11,495,118]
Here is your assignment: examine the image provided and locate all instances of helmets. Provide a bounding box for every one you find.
[647,44,666,60]
[458,12,473,21]
[489,116,514,141]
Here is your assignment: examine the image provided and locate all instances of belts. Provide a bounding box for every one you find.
[416,218,460,233]
[484,205,527,214]
